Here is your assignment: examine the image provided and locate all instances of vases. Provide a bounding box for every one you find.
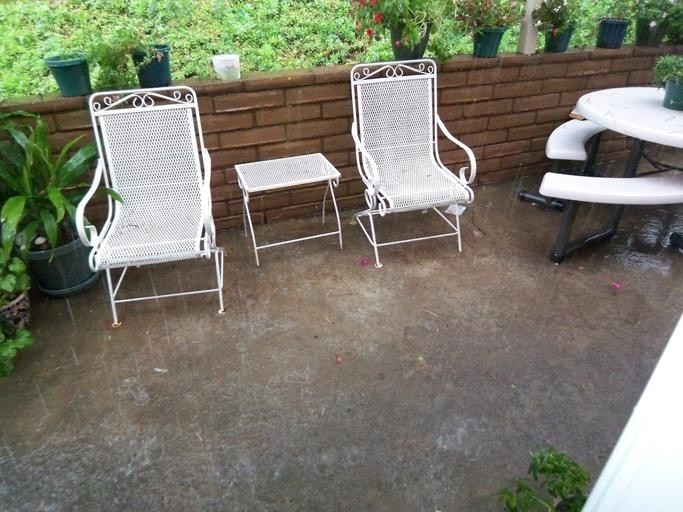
[42,52,92,97]
[132,45,173,88]
[389,20,433,63]
[473,27,507,59]
[543,21,576,53]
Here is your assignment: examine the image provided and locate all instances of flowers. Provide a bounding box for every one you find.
[530,0,582,36]
[349,1,454,50]
[454,1,528,32]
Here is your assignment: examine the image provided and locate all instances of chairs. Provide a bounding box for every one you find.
[350,58,478,269]
[73,85,227,329]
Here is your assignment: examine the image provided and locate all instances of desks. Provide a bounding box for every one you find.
[234,151,345,267]
[574,88,683,259]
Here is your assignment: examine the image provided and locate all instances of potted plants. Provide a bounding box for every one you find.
[595,0,631,50]
[0,257,34,378]
[632,1,674,48]
[0,110,124,299]
[650,55,683,111]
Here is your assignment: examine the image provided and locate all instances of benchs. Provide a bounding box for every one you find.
[518,117,609,212]
[538,171,683,264]
